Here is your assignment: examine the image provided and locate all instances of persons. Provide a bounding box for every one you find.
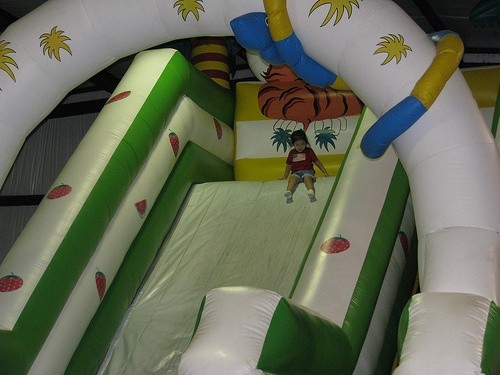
[278,130,331,200]
